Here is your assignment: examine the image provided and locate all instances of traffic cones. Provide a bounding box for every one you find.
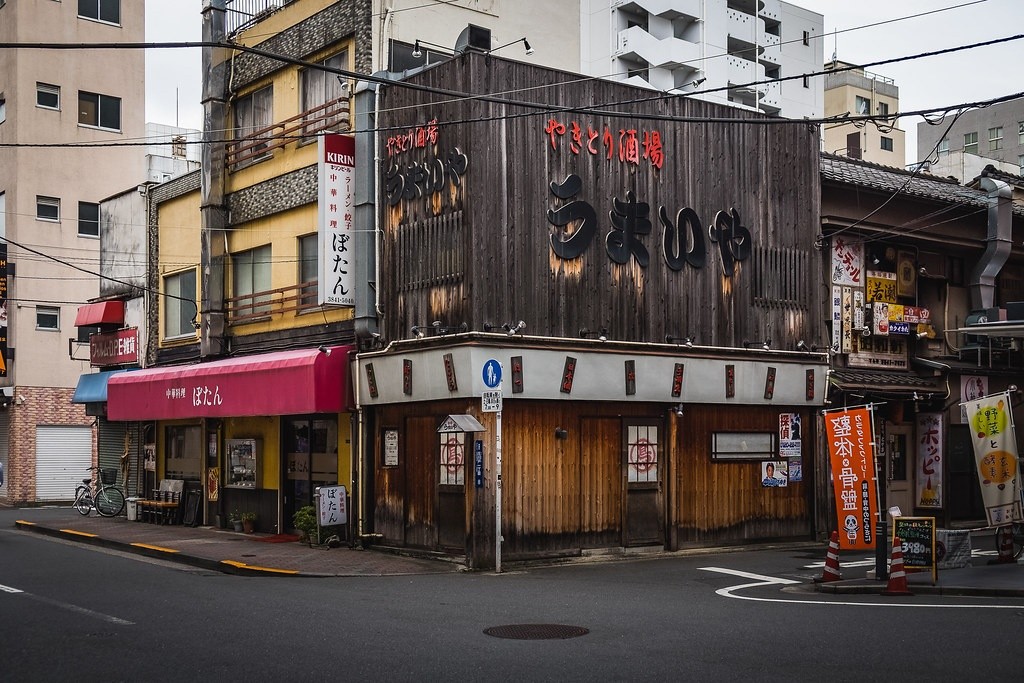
[883,536,919,597]
[813,531,843,583]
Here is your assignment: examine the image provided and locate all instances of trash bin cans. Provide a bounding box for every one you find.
[124,498,147,521]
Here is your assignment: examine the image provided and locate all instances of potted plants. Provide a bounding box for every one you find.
[229,512,256,533]
[292,506,322,549]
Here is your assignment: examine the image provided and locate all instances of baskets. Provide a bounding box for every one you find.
[100,468,117,484]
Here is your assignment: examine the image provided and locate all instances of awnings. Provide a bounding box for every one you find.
[74,301,124,326]
[108,345,352,421]
[71,368,140,403]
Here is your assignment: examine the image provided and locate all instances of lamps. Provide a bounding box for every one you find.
[337,75,387,90]
[851,326,871,336]
[665,78,706,95]
[555,426,568,440]
[484,37,535,66]
[483,321,527,336]
[579,327,607,341]
[411,321,469,339]
[318,342,346,357]
[797,340,839,356]
[871,255,879,265]
[412,39,464,66]
[672,402,684,417]
[741,338,772,350]
[664,333,695,347]
[916,332,928,340]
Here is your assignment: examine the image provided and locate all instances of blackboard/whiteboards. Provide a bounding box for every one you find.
[892,516,938,569]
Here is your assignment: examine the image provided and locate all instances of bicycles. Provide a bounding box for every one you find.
[995,522,1024,559]
[72,466,125,518]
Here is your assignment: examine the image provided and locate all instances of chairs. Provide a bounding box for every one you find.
[135,489,181,526]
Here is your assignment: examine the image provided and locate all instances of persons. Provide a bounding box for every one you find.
[792,418,800,440]
[763,463,779,487]
